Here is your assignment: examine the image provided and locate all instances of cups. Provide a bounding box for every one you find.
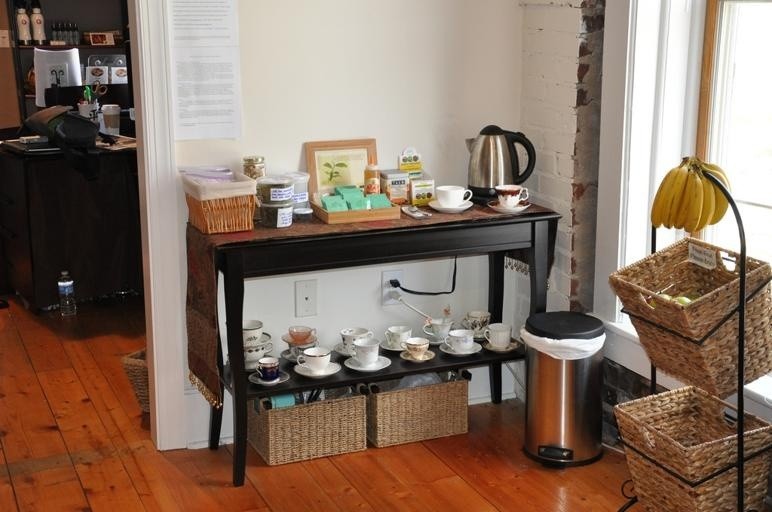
[494,186,530,210]
[401,338,431,360]
[298,348,332,375]
[347,338,382,368]
[423,321,454,342]
[243,320,264,346]
[289,342,319,360]
[485,324,511,349]
[445,330,475,353]
[256,358,282,384]
[241,343,274,363]
[436,186,473,209]
[340,328,375,353]
[385,326,413,350]
[461,311,492,339]
[289,326,317,342]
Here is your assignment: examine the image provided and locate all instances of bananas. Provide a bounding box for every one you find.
[651,153,689,228]
[696,156,732,192]
[684,162,704,233]
[696,159,731,226]
[662,159,693,229]
[674,162,695,228]
[692,160,716,232]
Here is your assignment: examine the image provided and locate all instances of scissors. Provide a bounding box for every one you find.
[91,80,108,103]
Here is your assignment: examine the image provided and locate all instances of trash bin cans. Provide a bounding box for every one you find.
[520,311,606,468]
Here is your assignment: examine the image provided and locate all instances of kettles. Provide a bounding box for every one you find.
[465,124,537,208]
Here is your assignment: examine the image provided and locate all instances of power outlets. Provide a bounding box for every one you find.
[295,279,318,317]
[381,269,405,305]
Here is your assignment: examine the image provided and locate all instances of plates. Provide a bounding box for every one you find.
[486,201,532,213]
[248,371,290,388]
[439,342,482,358]
[282,344,322,363]
[429,340,444,345]
[473,335,486,342]
[401,351,436,364]
[294,363,342,378]
[240,333,272,348]
[334,344,357,358]
[429,200,474,213]
[401,206,433,219]
[344,356,392,373]
[281,334,316,347]
[226,354,274,371]
[483,337,519,354]
[381,341,407,352]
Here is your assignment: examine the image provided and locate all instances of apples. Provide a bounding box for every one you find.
[672,296,692,306]
[650,294,673,308]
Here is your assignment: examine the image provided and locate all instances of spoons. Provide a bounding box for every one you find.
[409,205,429,218]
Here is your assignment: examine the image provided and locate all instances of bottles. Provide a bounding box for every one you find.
[58,22,64,43]
[364,155,381,194]
[72,23,80,45]
[52,21,58,43]
[30,10,47,44]
[65,22,70,42]
[57,269,77,318]
[18,7,31,46]
[244,155,266,220]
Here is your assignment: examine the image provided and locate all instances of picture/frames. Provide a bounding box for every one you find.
[304,138,377,203]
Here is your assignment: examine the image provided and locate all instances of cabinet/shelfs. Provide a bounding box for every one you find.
[6,0,136,138]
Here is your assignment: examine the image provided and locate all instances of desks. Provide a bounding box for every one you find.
[0,134,144,317]
